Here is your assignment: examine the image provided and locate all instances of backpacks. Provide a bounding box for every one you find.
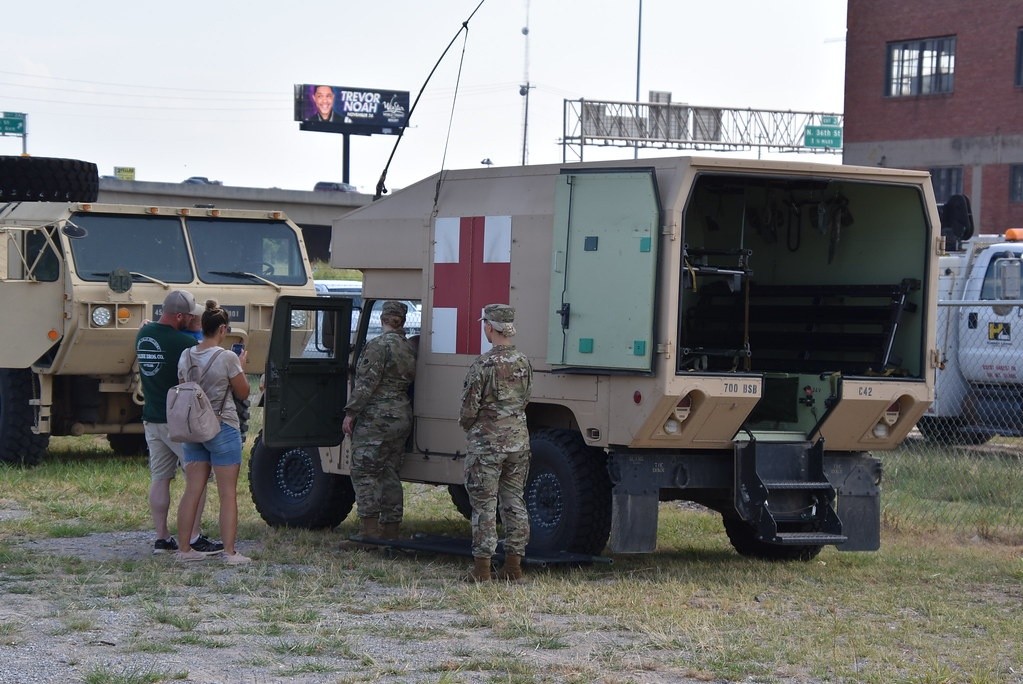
[166,348,231,443]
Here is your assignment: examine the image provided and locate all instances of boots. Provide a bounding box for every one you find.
[490,555,522,580]
[459,558,490,583]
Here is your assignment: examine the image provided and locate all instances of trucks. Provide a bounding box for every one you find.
[910,191,1023,446]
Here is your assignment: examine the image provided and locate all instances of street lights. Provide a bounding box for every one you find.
[519,85,529,165]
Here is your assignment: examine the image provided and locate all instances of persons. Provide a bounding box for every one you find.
[135,290,251,567]
[309,85,354,124]
[457,303,533,582]
[342,301,418,550]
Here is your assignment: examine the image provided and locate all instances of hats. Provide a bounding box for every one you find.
[162,290,205,315]
[477,304,514,323]
[382,302,408,317]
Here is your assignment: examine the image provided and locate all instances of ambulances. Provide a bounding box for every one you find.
[245,158,943,567]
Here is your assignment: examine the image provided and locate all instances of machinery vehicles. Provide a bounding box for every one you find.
[0,153,317,462]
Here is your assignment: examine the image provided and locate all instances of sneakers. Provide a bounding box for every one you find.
[190,534,225,555]
[153,537,179,555]
[176,548,206,563]
[222,551,251,564]
[349,516,401,540]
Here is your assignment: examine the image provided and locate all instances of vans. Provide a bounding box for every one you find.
[303,280,421,358]
